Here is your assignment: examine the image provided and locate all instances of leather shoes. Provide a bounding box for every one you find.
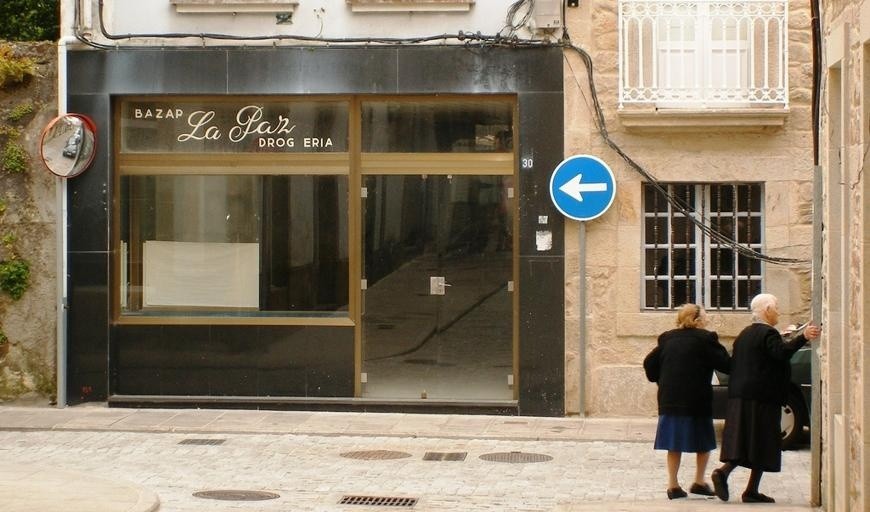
[742,491,775,503]
[711,469,729,501]
[667,486,687,500]
[690,482,715,496]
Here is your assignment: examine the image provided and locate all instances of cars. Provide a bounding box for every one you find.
[63,127,92,160]
[710,318,814,451]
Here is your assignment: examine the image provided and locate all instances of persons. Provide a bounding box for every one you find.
[711,293,820,502]
[643,302,733,501]
